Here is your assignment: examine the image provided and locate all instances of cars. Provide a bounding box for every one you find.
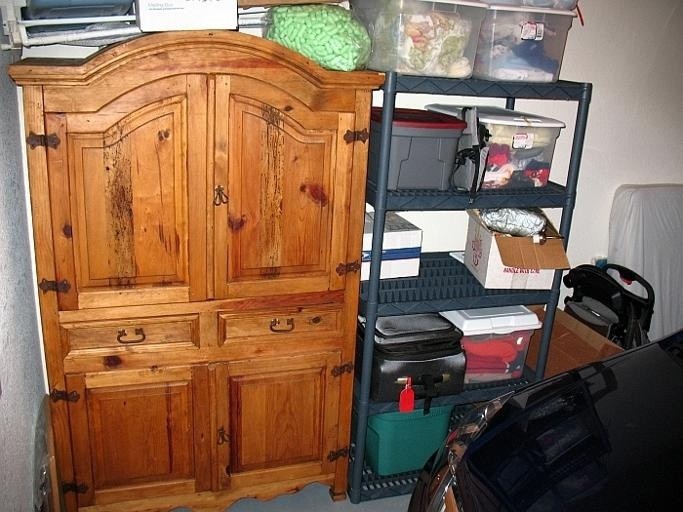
[407,328,683,512]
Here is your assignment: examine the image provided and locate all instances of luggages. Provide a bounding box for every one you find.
[355,311,468,402]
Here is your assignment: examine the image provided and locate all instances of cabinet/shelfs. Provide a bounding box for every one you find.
[3,30,388,511]
[346,69,593,503]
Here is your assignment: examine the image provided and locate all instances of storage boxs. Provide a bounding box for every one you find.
[524,306,625,381]
[462,207,571,292]
[367,104,463,190]
[361,212,424,284]
[473,1,578,84]
[439,305,543,385]
[348,2,487,80]
[135,0,240,33]
[365,405,457,477]
[424,102,566,193]
